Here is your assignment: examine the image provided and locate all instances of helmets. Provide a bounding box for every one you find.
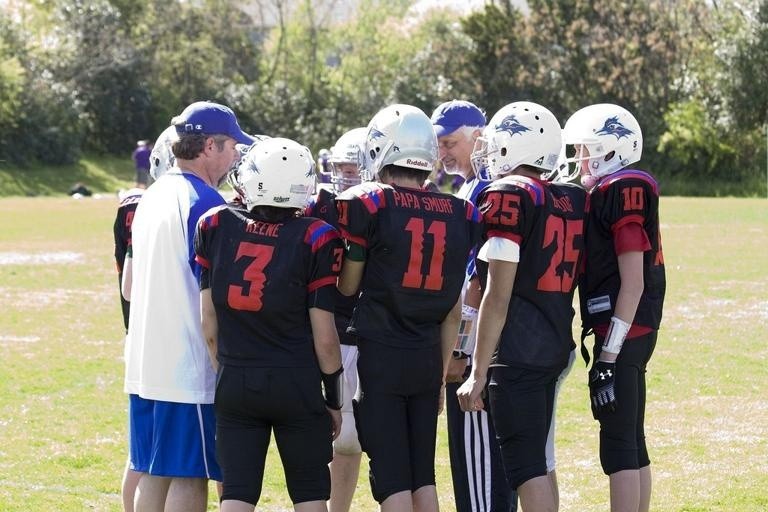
[362,103,440,185]
[469,101,564,184]
[237,137,318,214]
[148,125,181,182]
[557,103,643,188]
[223,134,273,206]
[325,126,370,197]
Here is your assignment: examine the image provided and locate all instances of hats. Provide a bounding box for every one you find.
[429,99,487,138]
[174,101,258,146]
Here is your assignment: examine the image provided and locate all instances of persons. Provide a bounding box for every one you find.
[112,98,667,512]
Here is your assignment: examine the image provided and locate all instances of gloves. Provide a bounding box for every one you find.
[586,359,618,421]
